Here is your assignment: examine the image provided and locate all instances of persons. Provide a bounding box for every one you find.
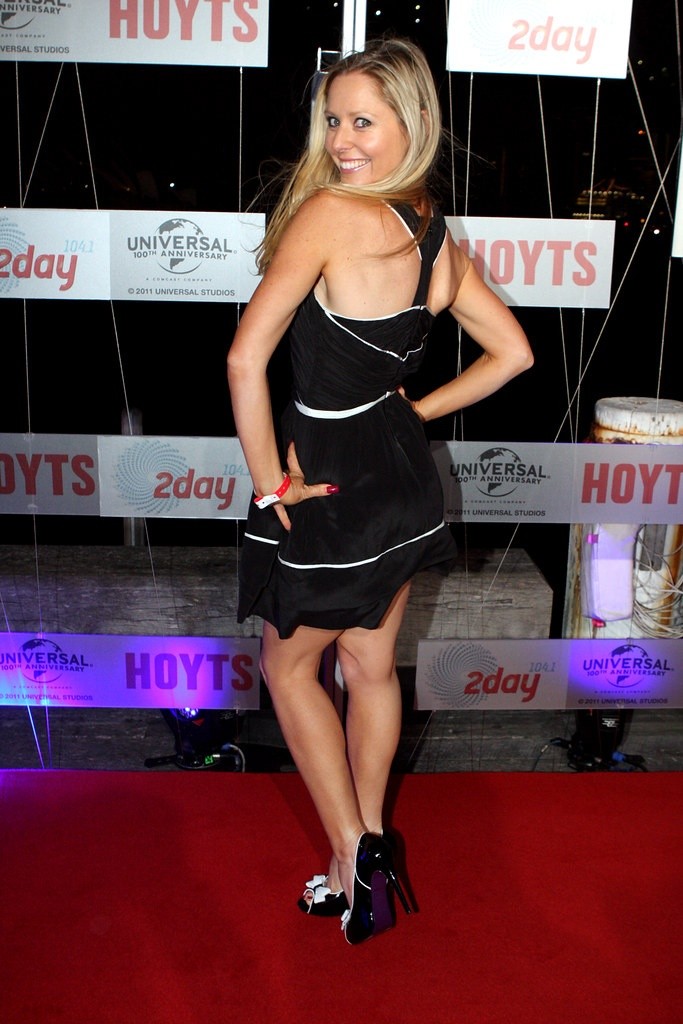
[227,40,534,944]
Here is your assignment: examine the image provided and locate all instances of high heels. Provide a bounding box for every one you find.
[299,830,397,916]
[340,831,411,945]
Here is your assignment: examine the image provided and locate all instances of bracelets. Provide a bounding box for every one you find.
[253,472,291,510]
